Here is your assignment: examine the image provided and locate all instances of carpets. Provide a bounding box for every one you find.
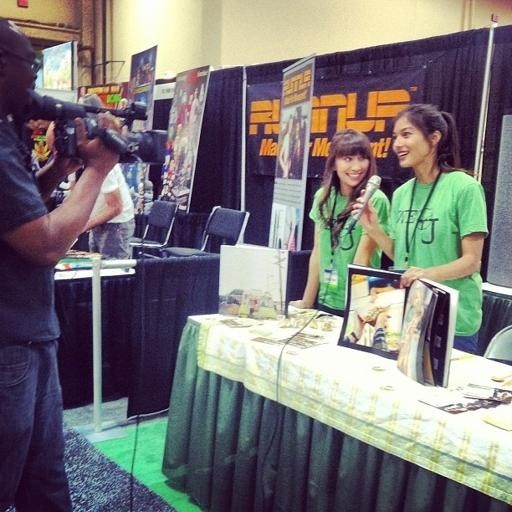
[91,418,204,512]
[6,421,176,512]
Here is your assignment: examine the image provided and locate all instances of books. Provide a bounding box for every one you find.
[338,261,459,390]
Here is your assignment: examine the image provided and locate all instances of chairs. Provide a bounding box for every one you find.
[483,323,511,367]
[128,200,180,259]
[162,206,250,258]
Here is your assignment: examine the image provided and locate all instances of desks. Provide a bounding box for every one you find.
[160,305,512,511]
[54,251,135,411]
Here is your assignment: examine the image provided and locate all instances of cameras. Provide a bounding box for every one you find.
[24,90,171,170]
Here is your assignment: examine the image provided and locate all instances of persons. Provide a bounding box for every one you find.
[351,104,488,355]
[46,113,135,261]
[0,17,128,512]
[288,129,391,318]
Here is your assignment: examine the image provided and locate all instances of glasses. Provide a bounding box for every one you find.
[3,49,44,73]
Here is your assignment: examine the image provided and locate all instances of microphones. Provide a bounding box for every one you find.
[344,174,382,234]
